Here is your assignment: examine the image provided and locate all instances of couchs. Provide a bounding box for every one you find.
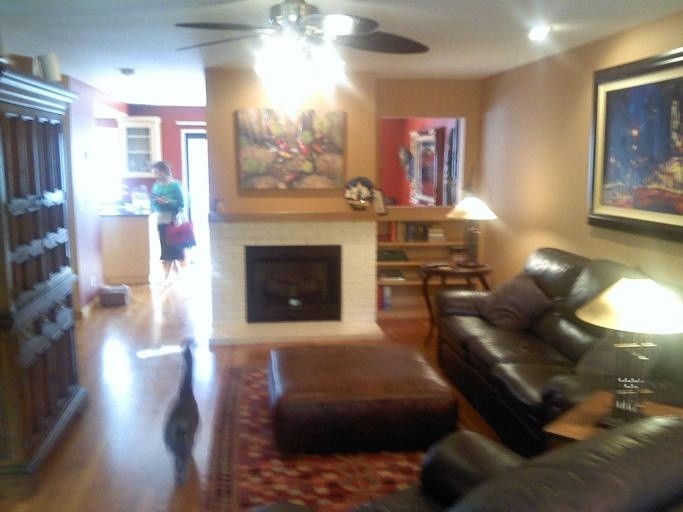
[435,247,674,450]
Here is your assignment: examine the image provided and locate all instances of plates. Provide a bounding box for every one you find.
[343,177,377,212]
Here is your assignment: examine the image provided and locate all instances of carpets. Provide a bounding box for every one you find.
[209,363,429,509]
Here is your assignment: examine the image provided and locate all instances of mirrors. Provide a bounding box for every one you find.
[377,117,467,209]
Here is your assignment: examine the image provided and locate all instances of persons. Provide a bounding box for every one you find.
[149,158,190,278]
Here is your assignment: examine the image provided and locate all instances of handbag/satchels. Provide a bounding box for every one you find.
[163,210,196,250]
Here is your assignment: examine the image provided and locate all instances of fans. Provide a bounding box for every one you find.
[176,0,430,56]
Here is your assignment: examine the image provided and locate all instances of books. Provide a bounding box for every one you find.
[378,219,446,244]
[377,285,392,310]
[376,248,409,261]
[378,267,406,281]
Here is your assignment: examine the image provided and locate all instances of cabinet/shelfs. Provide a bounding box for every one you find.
[124,115,162,178]
[377,218,469,287]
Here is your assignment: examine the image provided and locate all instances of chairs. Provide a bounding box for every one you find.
[356,428,683,511]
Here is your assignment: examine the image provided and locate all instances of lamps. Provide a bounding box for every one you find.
[446,195,498,267]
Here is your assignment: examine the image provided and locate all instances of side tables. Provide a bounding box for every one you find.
[418,264,494,344]
[545,389,683,456]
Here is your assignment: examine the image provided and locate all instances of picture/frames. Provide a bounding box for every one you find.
[586,44,683,244]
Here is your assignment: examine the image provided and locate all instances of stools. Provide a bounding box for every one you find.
[269,341,458,452]
